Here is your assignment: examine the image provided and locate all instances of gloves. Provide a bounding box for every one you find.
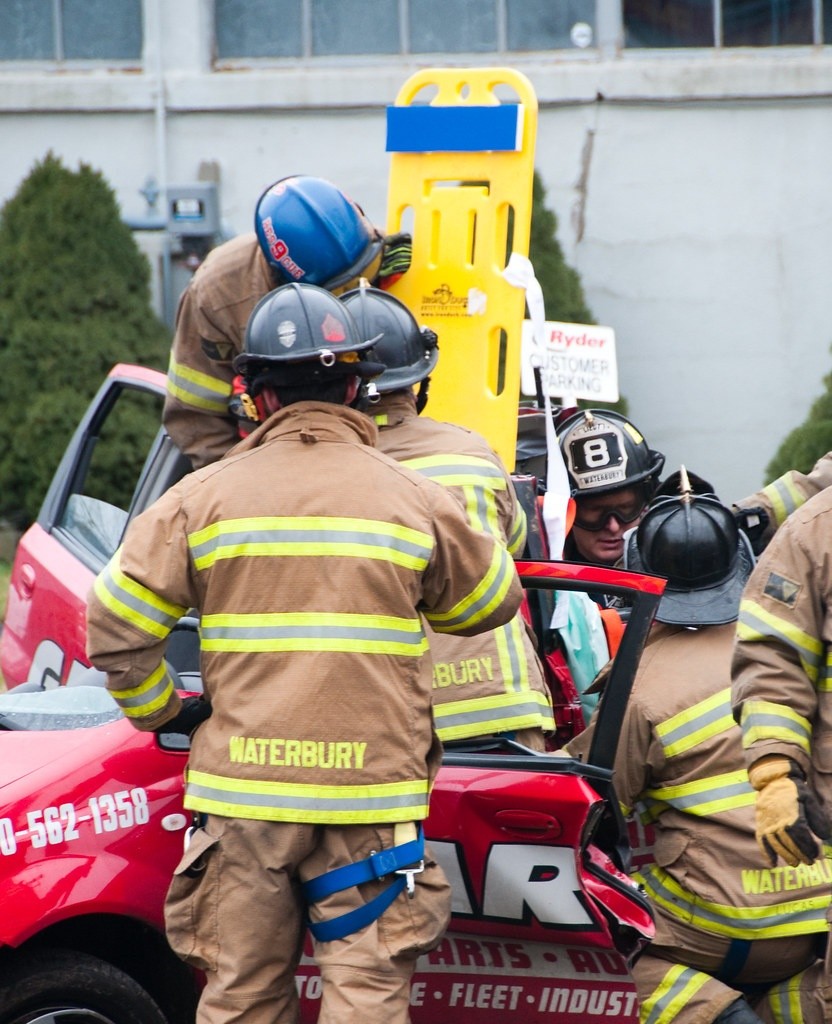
[748,754,831,868]
[149,692,212,736]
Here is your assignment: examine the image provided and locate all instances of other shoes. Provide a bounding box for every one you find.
[712,997,764,1024]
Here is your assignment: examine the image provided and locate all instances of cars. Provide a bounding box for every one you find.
[0,364,666,1023]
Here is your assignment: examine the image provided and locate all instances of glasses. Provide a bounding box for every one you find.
[572,481,651,531]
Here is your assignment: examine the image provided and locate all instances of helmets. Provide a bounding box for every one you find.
[623,463,759,627]
[231,281,385,377]
[254,173,382,288]
[544,409,664,496]
[338,277,439,397]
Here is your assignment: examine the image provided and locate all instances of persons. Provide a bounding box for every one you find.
[343,279,557,751]
[556,409,665,610]
[732,484,832,1024]
[87,283,523,1024]
[162,176,832,1024]
